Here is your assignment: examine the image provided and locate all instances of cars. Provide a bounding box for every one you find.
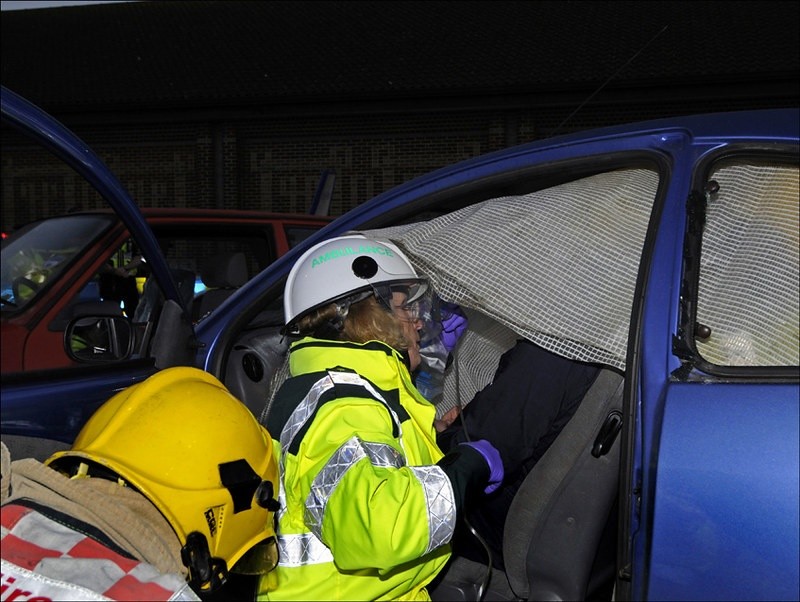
[0,85,800,602]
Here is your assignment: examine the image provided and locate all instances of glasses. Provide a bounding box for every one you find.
[388,302,421,324]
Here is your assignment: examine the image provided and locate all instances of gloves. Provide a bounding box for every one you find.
[424,298,469,353]
[460,439,503,494]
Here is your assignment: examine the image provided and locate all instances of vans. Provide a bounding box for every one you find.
[0,210,340,388]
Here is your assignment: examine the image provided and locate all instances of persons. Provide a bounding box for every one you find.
[436,338,603,569]
[14,243,151,360]
[257,234,503,602]
[0,366,282,602]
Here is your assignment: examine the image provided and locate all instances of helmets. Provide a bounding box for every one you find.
[44,366,280,591]
[279,235,432,337]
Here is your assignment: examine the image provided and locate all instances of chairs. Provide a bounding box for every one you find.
[80,272,161,360]
[429,363,627,602]
